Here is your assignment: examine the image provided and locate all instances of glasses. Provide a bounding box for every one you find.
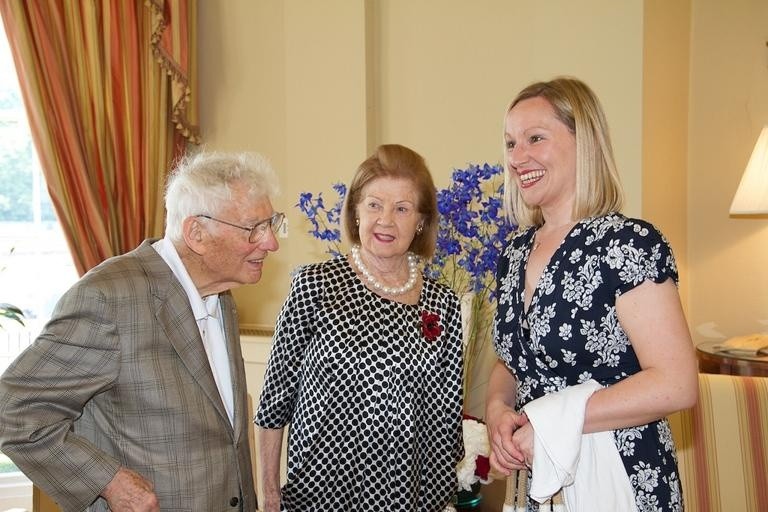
[197,212,287,245]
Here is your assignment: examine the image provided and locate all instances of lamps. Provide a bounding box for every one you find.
[728,125,768,220]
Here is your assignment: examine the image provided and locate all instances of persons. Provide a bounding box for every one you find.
[485,78,699,512]
[0,149,277,512]
[253,144,465,512]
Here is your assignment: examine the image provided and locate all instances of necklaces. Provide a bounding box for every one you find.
[351,245,418,296]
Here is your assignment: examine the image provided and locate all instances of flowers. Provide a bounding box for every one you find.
[423,159,514,300]
[417,309,443,342]
[296,182,346,255]
[454,409,493,494]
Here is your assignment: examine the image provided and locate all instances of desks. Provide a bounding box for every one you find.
[695,336,768,376]
